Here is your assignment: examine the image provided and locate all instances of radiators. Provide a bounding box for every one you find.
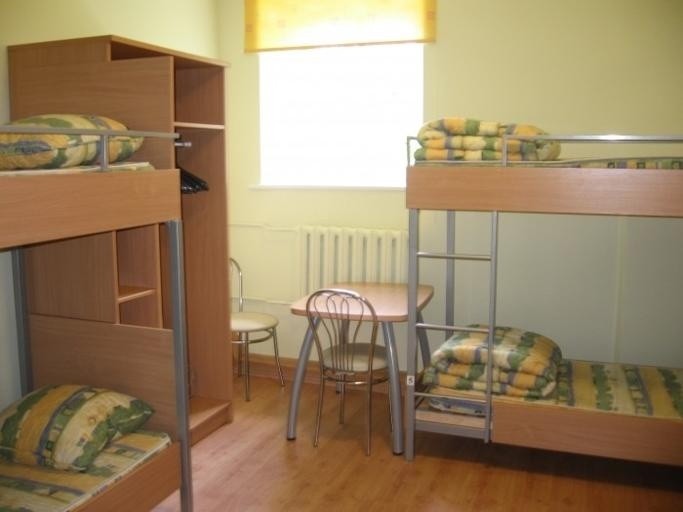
[299,230,406,303]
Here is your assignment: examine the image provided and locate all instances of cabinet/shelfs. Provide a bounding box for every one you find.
[8,35,234,448]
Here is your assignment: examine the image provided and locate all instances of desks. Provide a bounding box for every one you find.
[287,281,435,455]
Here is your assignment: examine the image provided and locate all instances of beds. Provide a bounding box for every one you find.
[405,134,680,468]
[0,125,191,506]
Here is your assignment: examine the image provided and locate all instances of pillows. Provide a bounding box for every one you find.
[1,111,146,170]
[0,382,154,472]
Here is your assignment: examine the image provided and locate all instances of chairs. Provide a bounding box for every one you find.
[228,257,286,403]
[306,288,393,456]
